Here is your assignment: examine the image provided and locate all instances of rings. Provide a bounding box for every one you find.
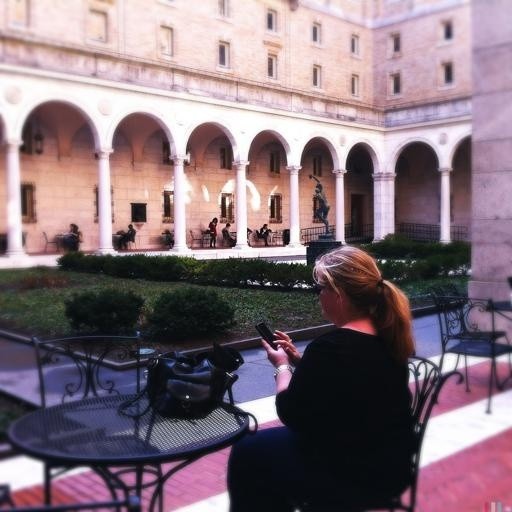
[287,338,293,343]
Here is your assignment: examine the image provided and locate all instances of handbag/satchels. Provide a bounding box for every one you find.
[147,346,244,419]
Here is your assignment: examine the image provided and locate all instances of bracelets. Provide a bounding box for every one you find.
[273,364,293,380]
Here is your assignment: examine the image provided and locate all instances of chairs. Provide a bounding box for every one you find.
[27,329,161,510]
[40,228,289,256]
[429,293,512,415]
[394,357,464,512]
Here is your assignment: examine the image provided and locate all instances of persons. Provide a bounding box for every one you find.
[64,216,273,252]
[309,171,331,236]
[225,244,419,512]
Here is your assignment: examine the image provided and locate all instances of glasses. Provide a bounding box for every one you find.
[312,284,325,294]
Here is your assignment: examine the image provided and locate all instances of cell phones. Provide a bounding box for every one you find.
[256,321,281,351]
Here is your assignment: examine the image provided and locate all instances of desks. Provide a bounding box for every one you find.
[7,392,259,512]
[484,299,512,322]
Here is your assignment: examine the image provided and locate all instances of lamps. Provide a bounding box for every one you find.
[34,128,44,154]
